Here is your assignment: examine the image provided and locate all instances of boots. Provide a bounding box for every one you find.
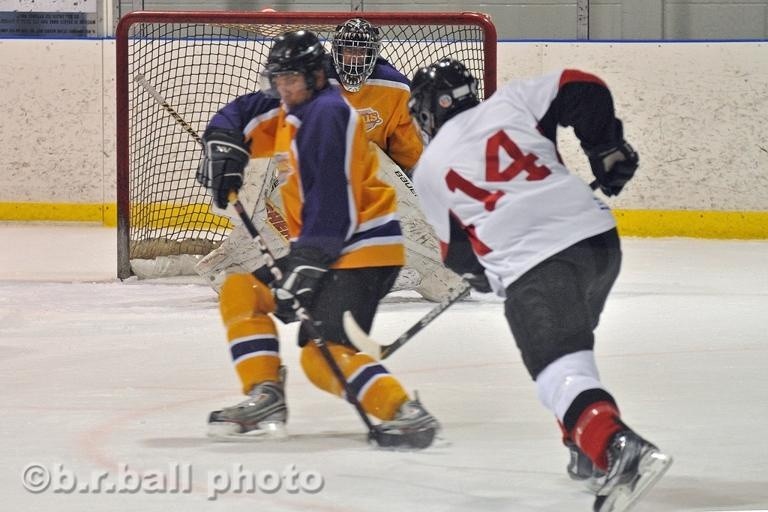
[210,364,287,429]
[557,407,660,510]
[370,400,437,449]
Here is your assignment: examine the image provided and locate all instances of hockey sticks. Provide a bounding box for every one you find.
[228,191,435,449]
[341,179,601,359]
[135,74,422,292]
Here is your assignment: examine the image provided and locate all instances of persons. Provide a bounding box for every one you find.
[196,31,444,433]
[197,16,470,303]
[407,57,661,511]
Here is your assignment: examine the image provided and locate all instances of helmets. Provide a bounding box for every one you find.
[332,18,379,87]
[264,30,328,77]
[406,57,480,143]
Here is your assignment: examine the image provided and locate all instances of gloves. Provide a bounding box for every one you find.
[580,119,639,196]
[270,248,327,324]
[463,271,493,293]
[202,127,249,210]
